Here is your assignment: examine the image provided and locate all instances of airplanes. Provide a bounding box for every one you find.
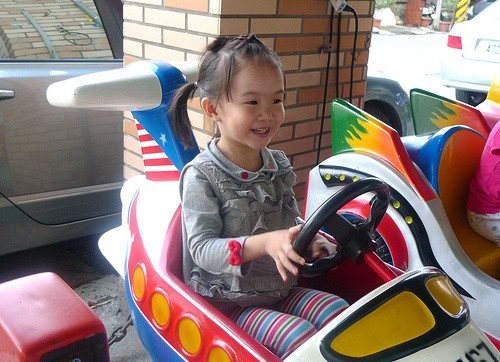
[47,58,500,362]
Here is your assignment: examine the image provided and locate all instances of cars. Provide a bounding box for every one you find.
[445,0,500,105]
[1,0,415,260]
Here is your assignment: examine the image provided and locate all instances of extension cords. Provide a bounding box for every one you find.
[329,0,348,13]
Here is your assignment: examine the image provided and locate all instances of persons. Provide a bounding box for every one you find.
[467,118,500,249]
[164,33,350,362]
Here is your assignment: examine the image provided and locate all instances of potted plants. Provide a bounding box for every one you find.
[420,4,436,27]
[438,8,454,33]
